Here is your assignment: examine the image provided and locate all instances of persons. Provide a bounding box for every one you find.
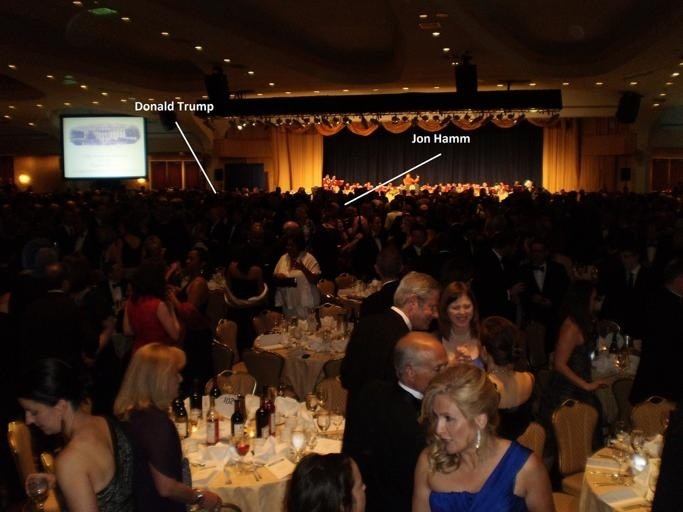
[0,172,683,511]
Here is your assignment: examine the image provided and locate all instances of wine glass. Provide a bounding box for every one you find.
[229,432,249,472]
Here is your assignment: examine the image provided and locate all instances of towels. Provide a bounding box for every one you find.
[182,394,313,468]
[352,279,396,298]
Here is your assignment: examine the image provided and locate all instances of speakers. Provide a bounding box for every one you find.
[614,94,641,124]
[454,63,477,91]
[205,73,230,98]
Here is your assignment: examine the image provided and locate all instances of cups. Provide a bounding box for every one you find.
[23,473,50,511]
[276,388,343,462]
[610,411,670,503]
[7,422,24,452]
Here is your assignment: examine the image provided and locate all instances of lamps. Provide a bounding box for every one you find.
[228,109,560,131]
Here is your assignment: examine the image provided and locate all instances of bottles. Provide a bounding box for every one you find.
[607,326,630,372]
[280,307,354,350]
[171,376,275,445]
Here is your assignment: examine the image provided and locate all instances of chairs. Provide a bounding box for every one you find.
[550,398,599,496]
[525,320,547,368]
[608,376,633,432]
[516,421,579,512]
[205,272,357,418]
[6,420,45,488]
[630,395,676,435]
